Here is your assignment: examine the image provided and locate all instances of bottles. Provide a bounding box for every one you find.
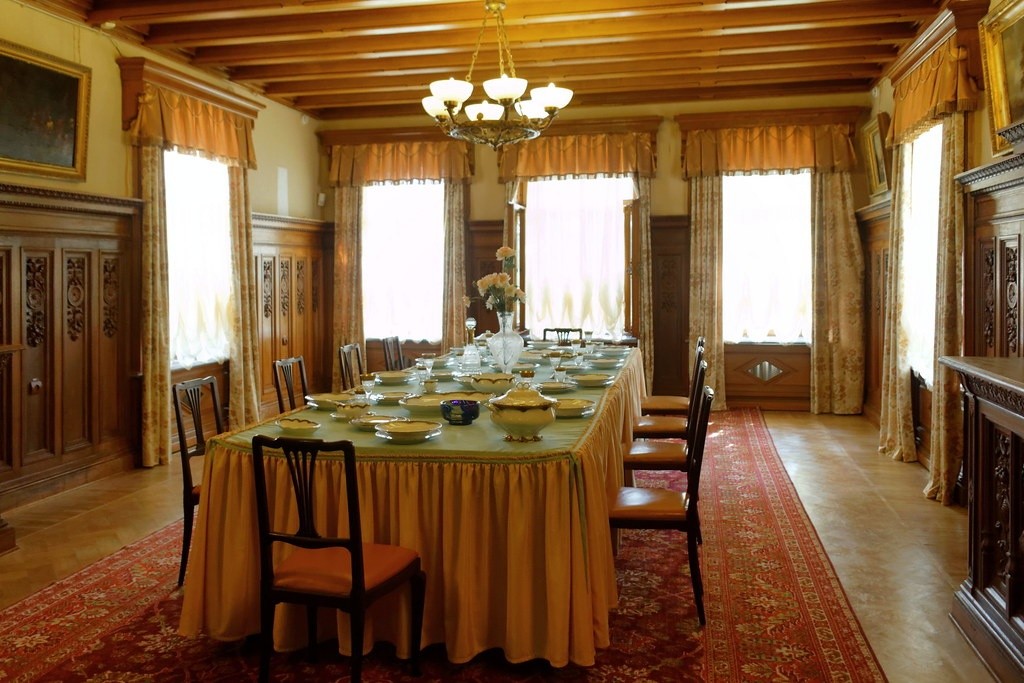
[462,317,481,371]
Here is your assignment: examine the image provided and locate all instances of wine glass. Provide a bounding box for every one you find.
[418,352,435,384]
[572,339,582,355]
[550,353,562,378]
[361,377,377,416]
[555,368,566,383]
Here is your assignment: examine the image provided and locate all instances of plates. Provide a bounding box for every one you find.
[489,362,540,373]
[522,345,629,416]
[371,371,412,382]
[276,391,443,443]
[453,376,472,388]
[450,346,461,354]
[398,391,496,416]
[416,358,449,367]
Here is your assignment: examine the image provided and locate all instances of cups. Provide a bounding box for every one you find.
[586,342,593,355]
[419,379,439,392]
[585,332,592,341]
[519,370,535,387]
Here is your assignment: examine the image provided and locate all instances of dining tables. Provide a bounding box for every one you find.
[177,339,651,683]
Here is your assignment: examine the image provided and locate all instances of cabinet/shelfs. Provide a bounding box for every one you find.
[935,353,1024,683]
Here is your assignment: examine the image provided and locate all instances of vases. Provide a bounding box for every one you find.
[487,310,524,377]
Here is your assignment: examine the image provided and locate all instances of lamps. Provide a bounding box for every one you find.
[422,0,576,153]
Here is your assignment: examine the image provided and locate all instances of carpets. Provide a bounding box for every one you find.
[0,404,893,683]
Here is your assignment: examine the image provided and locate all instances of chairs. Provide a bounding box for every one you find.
[633,346,706,439]
[270,354,311,415]
[379,335,406,371]
[170,375,236,589]
[641,335,707,416]
[340,344,366,390]
[608,383,716,629]
[542,327,584,340]
[622,357,710,543]
[248,435,433,683]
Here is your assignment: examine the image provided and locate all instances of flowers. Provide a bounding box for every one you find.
[462,242,532,329]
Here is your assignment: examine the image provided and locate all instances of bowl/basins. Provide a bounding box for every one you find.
[526,341,557,349]
[442,399,480,426]
[472,372,516,397]
[474,329,495,361]
[486,385,559,441]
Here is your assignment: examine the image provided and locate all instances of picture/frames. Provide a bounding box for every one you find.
[0,37,93,182]
